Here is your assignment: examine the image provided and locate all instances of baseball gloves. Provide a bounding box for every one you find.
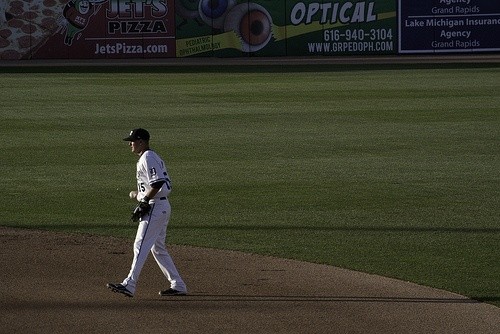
[131,202,151,222]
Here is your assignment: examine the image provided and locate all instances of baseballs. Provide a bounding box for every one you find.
[129,191,136,199]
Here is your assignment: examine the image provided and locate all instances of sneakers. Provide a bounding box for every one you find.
[159,288,187,295]
[106,283,133,297]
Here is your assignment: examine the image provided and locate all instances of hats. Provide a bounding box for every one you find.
[123,128,149,142]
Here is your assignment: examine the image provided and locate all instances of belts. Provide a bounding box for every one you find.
[160,197,166,200]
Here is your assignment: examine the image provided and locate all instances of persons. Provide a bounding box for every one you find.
[106,128,187,297]
